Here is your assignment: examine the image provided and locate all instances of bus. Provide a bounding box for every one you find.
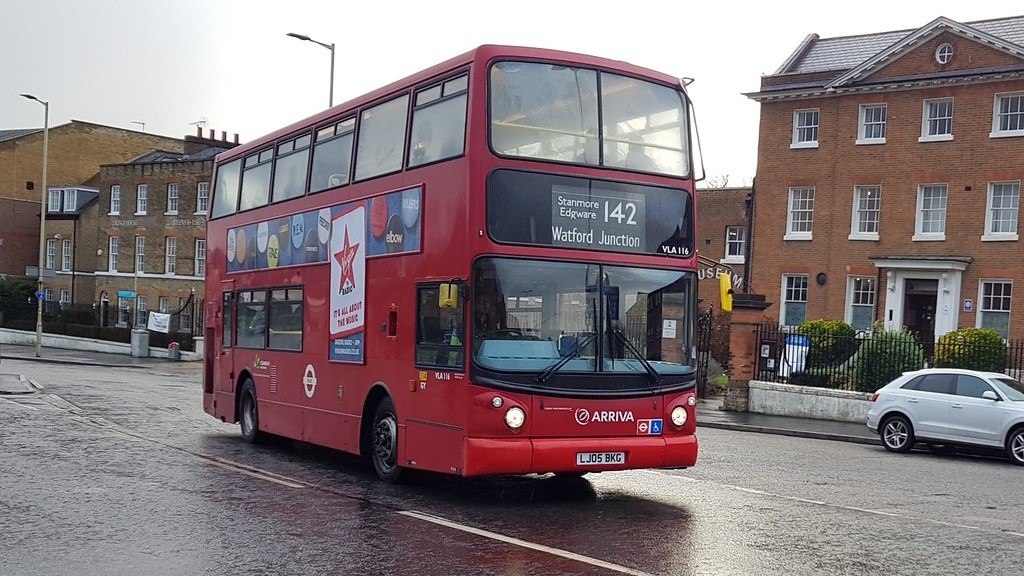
[204,44,706,484]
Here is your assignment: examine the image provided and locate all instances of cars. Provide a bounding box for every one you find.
[866,369,1024,464]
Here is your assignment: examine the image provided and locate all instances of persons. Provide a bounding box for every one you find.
[572,128,663,174]
[259,298,295,350]
[474,289,504,355]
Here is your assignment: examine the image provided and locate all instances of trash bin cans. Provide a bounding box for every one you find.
[130,328,152,358]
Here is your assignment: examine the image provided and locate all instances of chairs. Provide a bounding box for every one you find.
[233,298,302,351]
[226,139,456,213]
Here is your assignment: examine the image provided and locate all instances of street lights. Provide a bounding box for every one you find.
[287,32,336,110]
[19,93,48,357]
